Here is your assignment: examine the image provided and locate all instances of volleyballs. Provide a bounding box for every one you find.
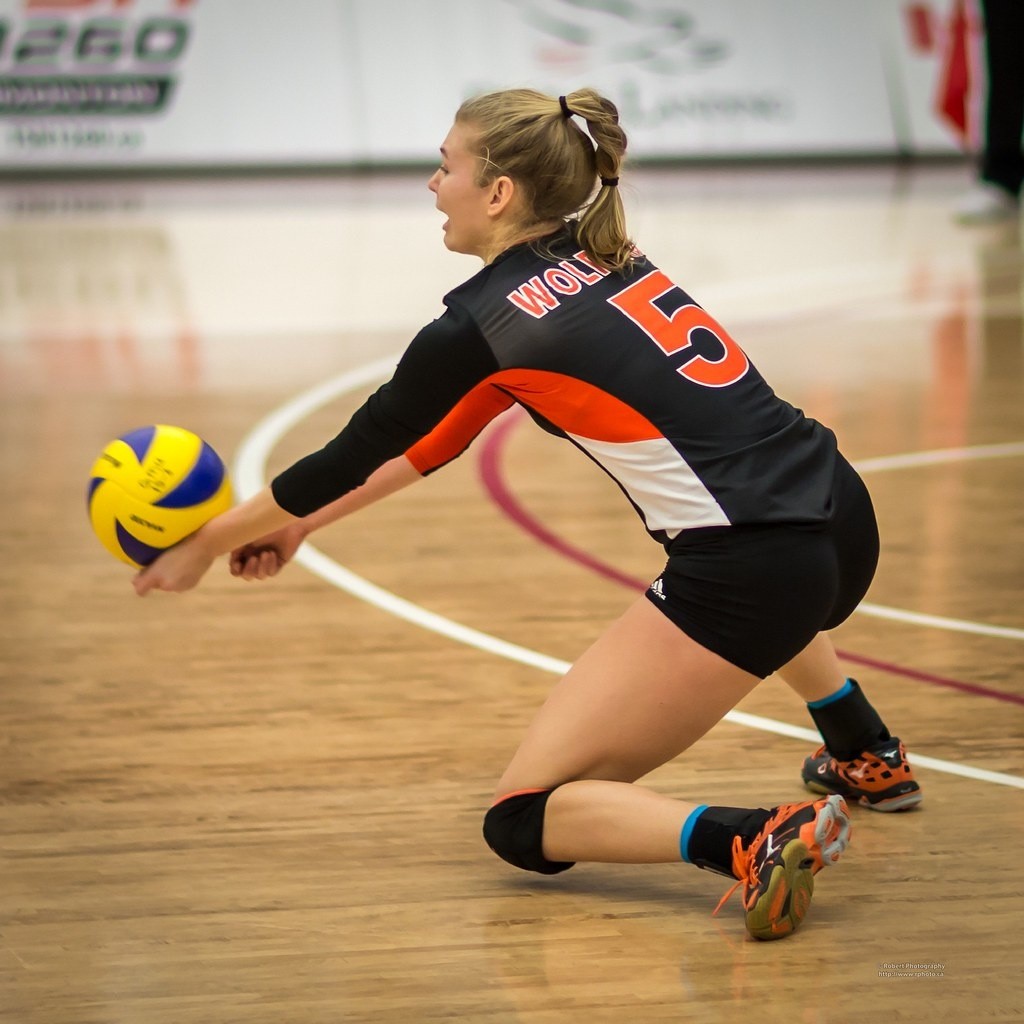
[85,424,236,568]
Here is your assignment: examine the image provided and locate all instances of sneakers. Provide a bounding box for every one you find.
[801,737,923,814]
[713,793,853,941]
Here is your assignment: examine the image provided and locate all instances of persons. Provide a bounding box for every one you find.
[135,89,924,942]
